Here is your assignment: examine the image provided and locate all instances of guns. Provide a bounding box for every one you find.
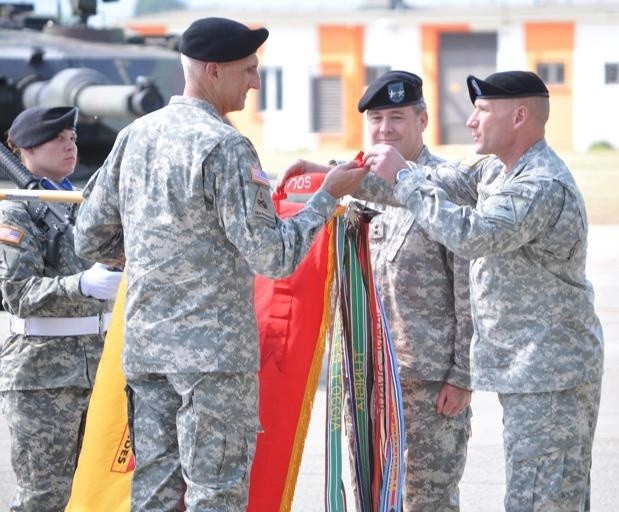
[0,141,120,302]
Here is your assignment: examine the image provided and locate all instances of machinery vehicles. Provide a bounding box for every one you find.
[1,0,187,191]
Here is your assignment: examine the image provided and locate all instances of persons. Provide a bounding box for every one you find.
[0,106,123,512]
[341,69,475,512]
[74,17,370,512]
[275,69,604,512]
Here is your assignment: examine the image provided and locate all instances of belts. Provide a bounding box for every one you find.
[9,313,112,336]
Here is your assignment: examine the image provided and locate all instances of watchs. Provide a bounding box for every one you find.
[395,169,410,184]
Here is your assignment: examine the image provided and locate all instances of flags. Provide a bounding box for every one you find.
[324,198,406,512]
[62,201,341,512]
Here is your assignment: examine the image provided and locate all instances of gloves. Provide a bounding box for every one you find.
[80,262,123,299]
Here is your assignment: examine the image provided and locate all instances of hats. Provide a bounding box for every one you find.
[9,106,78,149]
[358,70,423,113]
[179,18,269,63]
[467,70,549,105]
